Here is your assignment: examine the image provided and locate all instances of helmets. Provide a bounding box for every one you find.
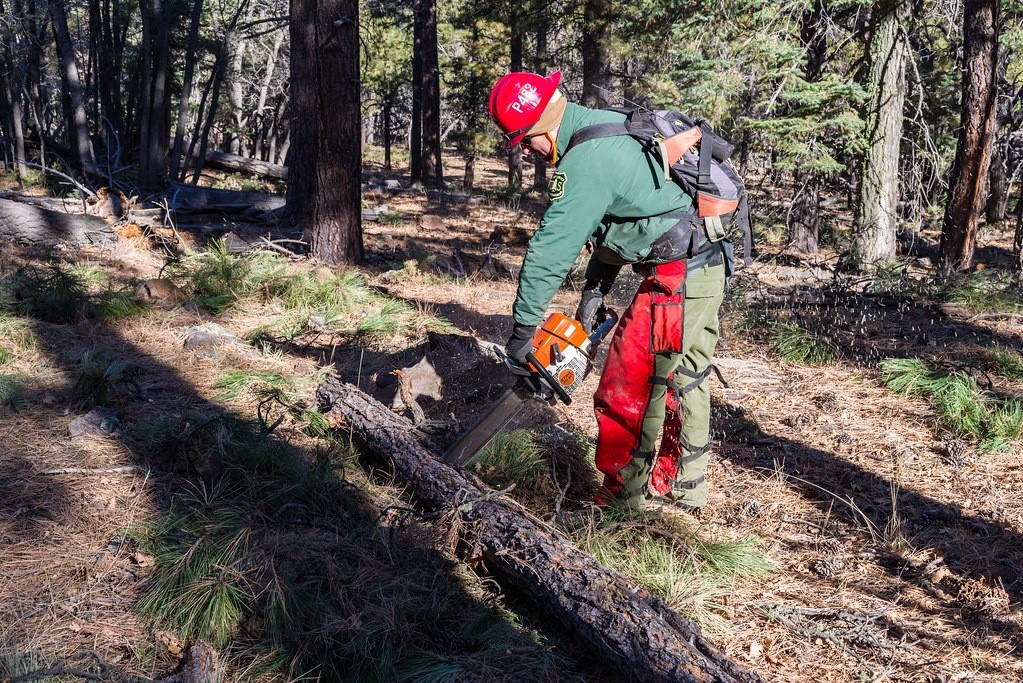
[485,71,562,148]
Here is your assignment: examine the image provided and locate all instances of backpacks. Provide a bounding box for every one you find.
[567,106,748,237]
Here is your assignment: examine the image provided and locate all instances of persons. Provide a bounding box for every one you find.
[487,70,730,518]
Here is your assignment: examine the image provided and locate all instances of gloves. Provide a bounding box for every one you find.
[504,321,537,366]
[574,287,602,333]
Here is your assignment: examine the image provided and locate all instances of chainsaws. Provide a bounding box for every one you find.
[441,300,618,472]
